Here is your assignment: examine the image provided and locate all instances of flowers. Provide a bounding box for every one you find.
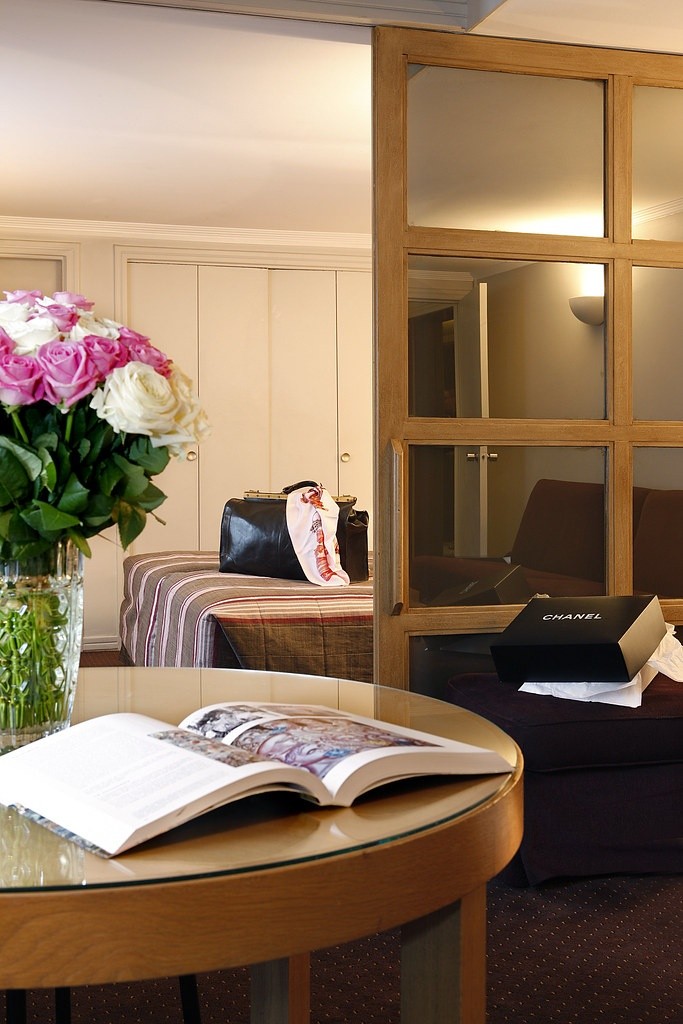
[0,288,215,559]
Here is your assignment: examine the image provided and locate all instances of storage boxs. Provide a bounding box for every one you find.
[431,560,535,607]
[489,589,667,683]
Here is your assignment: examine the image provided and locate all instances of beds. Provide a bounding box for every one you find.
[119,550,373,683]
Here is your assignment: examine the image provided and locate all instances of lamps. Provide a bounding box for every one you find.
[568,296,603,326]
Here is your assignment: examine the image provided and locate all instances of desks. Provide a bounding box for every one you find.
[0,666,525,1024]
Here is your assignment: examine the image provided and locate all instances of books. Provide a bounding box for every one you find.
[0,702,514,858]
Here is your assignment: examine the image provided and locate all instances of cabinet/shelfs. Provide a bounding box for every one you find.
[128,261,271,556]
[270,269,373,552]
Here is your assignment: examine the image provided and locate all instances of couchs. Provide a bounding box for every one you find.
[409,477,683,607]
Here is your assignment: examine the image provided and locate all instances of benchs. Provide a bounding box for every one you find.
[444,671,683,888]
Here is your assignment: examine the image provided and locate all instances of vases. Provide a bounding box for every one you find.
[0,537,84,757]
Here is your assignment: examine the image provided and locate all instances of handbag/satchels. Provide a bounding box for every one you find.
[218,482,369,581]
